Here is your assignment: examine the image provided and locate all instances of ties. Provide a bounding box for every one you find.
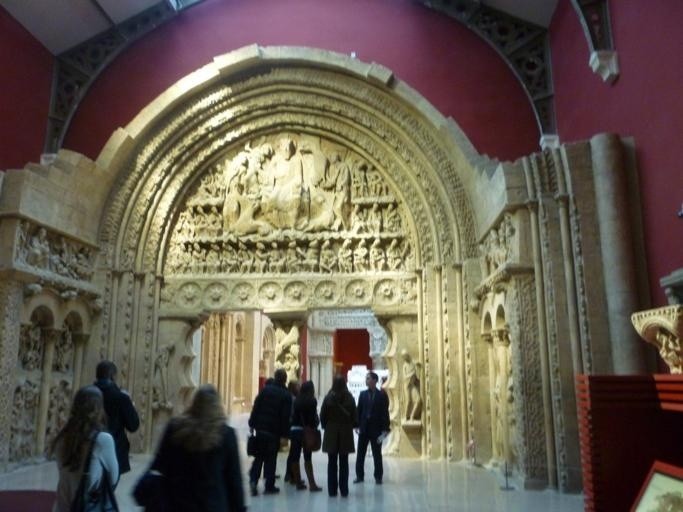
[369,391,374,401]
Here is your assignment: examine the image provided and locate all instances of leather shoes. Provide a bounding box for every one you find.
[376,478,383,484]
[353,478,364,483]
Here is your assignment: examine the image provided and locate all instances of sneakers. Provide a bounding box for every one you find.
[296,485,307,490]
[250,489,258,496]
[263,487,281,495]
[309,486,322,492]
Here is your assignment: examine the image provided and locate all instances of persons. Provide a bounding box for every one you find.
[478,214,517,466]
[283,355,298,386]
[150,340,176,409]
[163,134,409,275]
[8,219,92,463]
[400,349,420,421]
[131,382,248,512]
[45,385,119,512]
[244,367,390,497]
[93,360,139,474]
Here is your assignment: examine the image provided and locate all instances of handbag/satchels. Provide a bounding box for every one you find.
[302,425,322,452]
[68,470,123,512]
[131,467,170,510]
[247,434,258,456]
